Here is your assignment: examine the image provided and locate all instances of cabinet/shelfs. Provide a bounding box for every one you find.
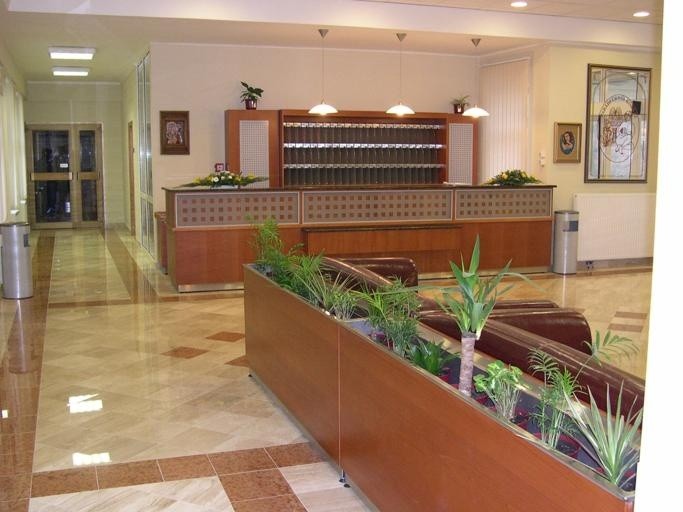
[279,107,448,187]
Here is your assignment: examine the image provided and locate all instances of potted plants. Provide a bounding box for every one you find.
[239,79,264,111]
[365,279,645,491]
[252,218,372,320]
[448,94,470,115]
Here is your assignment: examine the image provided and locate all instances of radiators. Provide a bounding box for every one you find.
[572,192,655,261]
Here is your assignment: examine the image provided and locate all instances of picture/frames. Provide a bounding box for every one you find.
[586,61,652,184]
[553,121,582,164]
[158,109,190,156]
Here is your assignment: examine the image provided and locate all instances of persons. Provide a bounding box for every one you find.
[562,133,574,154]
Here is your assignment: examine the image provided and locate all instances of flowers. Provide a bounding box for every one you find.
[479,166,544,187]
[181,168,270,188]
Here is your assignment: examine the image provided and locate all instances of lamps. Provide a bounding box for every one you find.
[307,29,340,117]
[48,46,96,61]
[384,33,414,117]
[460,37,491,120]
[49,66,90,77]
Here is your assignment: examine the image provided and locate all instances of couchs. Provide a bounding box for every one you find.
[318,256,558,315]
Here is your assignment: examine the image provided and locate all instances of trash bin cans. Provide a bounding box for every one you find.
[0,221,34,300]
[553,210,579,275]
